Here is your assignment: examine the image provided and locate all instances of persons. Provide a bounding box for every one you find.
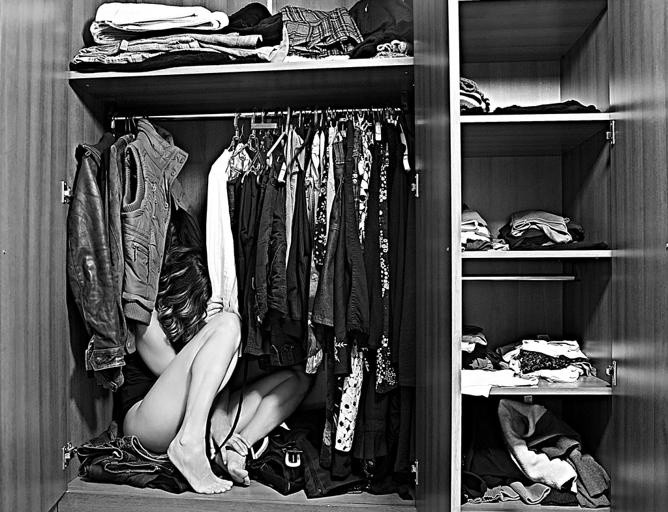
[115,219,312,495]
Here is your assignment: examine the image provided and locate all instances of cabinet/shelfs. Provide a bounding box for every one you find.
[1,1,419,512]
[445,0,667,510]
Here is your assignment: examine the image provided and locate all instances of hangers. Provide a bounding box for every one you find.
[111,111,150,134]
[224,106,401,183]
